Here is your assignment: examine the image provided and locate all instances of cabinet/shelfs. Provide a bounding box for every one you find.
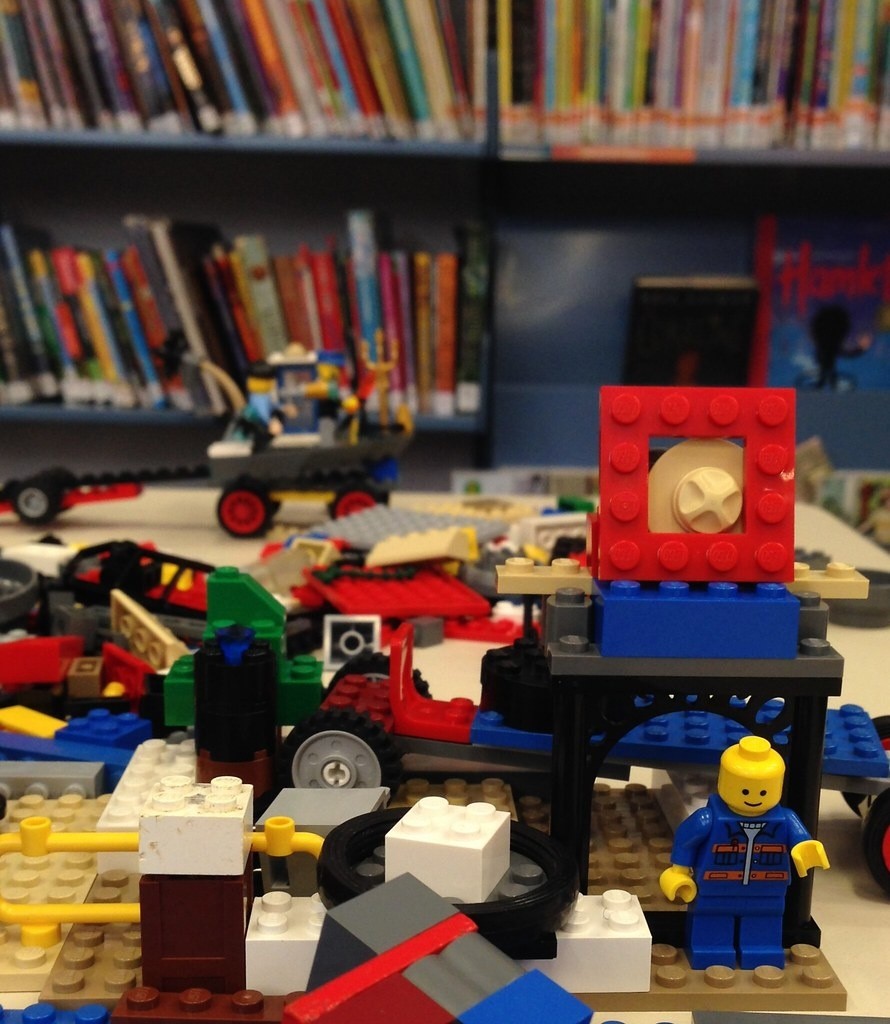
[0,0,890,469]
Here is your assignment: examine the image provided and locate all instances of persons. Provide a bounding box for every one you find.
[660,736,828,970]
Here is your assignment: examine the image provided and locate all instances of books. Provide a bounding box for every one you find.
[0,0,890,150]
[621,217,889,392]
[0,211,489,418]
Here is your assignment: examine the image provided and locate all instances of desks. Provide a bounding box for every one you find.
[0,485,890,1023]
